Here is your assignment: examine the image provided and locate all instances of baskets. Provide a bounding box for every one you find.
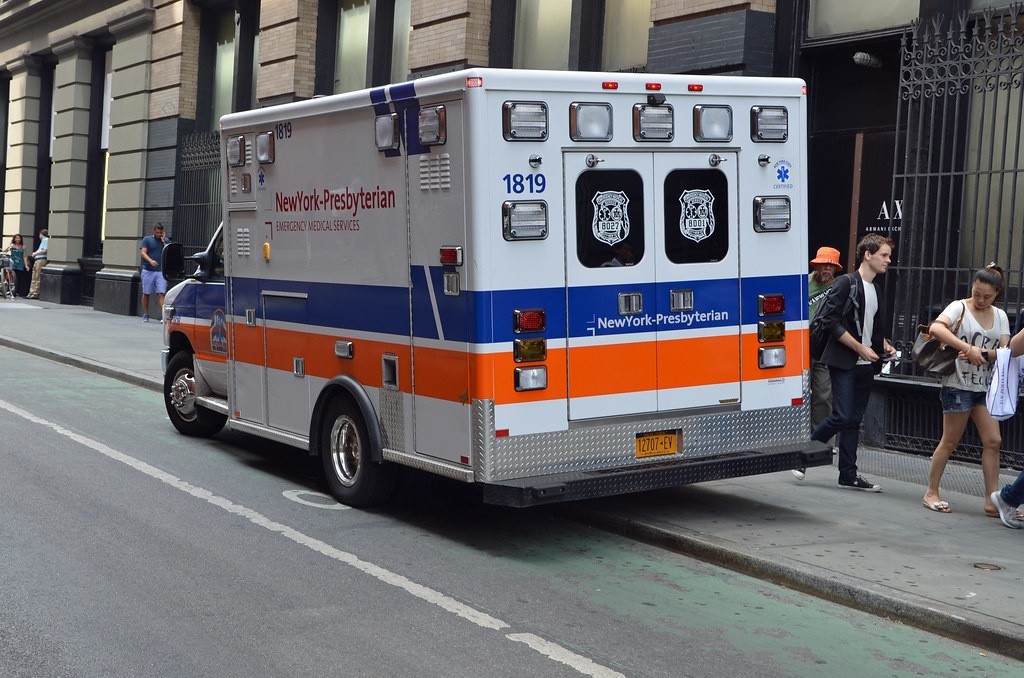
[0,254,10,266]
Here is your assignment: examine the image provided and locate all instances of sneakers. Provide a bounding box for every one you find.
[792,467,805,480]
[991,491,1021,529]
[838,475,882,492]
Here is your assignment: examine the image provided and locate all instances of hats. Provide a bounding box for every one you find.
[39,229,48,236]
[810,247,842,272]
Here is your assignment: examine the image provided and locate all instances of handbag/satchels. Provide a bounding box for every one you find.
[910,301,964,375]
[809,273,856,360]
[985,336,1020,421]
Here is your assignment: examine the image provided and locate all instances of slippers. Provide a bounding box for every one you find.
[922,500,951,513]
[986,509,1024,520]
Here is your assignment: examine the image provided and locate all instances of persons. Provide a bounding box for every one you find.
[991,308,1024,528]
[26,229,49,299]
[793,232,896,493]
[140,222,170,323]
[808,246,843,452]
[923,263,1024,520]
[3,233,30,297]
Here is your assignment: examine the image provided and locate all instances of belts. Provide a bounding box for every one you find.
[35,257,47,260]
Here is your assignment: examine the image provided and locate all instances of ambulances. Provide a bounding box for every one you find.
[158,68,837,512]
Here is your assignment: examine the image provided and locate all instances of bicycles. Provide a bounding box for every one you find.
[0,251,18,300]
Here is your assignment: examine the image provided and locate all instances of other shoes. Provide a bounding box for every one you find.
[25,293,31,298]
[142,314,148,322]
[29,294,39,299]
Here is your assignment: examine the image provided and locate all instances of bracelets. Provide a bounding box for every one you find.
[966,344,972,355]
